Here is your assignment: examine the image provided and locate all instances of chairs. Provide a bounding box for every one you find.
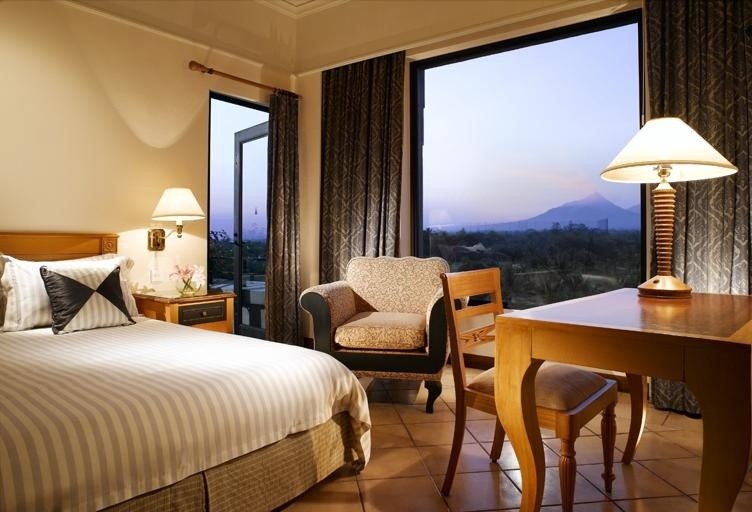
[440,268,617,510]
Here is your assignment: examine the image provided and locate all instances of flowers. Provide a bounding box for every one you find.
[170,264,204,294]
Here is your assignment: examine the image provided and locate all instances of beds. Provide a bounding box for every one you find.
[0,232,364,512]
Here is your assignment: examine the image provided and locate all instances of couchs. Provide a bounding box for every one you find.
[300,256,469,413]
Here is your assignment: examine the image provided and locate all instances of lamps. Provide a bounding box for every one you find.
[148,187,206,252]
[604,114,738,302]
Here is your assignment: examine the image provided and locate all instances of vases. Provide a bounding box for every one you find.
[168,274,202,297]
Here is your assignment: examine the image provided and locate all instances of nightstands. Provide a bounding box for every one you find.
[129,283,237,341]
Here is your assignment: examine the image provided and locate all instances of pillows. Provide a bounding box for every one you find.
[0,250,139,338]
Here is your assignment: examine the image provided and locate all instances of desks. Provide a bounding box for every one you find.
[490,284,752,511]
